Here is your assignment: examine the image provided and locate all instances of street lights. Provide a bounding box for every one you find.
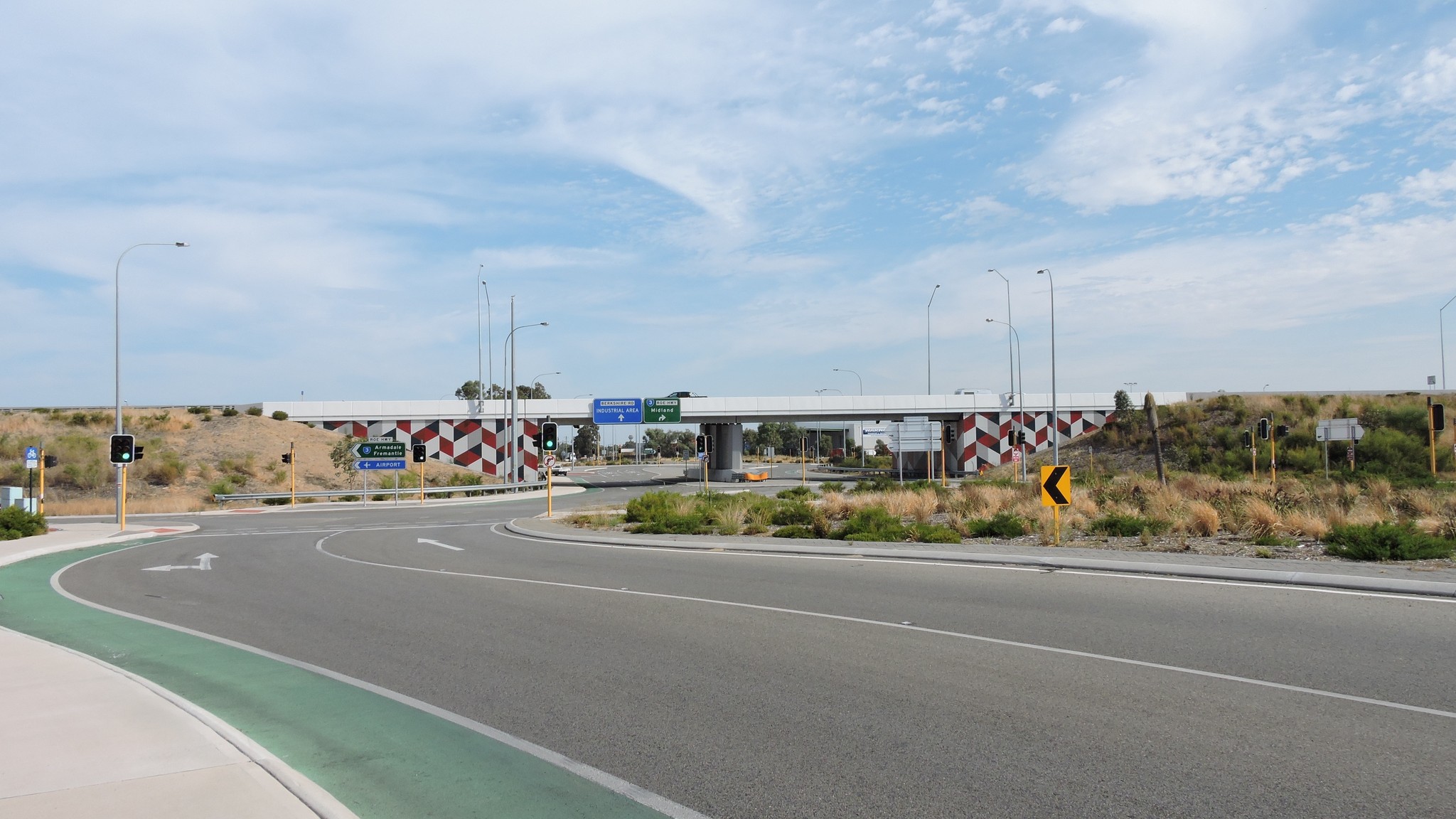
[803,260,1063,489]
[482,279,494,401]
[113,241,191,524]
[477,263,485,413]
[527,371,561,399]
[569,393,594,471]
[501,318,549,493]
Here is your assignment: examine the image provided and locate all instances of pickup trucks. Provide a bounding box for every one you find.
[665,391,709,398]
[546,464,571,476]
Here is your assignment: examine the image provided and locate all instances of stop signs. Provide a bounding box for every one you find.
[1347,447,1353,454]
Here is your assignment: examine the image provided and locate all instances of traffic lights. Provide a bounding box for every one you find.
[592,448,595,454]
[542,422,558,452]
[110,435,135,464]
[696,435,706,453]
[413,444,426,463]
[618,448,621,453]
[676,446,679,451]
[657,447,660,452]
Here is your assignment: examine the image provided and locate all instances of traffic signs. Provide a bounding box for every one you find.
[643,397,681,423]
[593,397,642,423]
